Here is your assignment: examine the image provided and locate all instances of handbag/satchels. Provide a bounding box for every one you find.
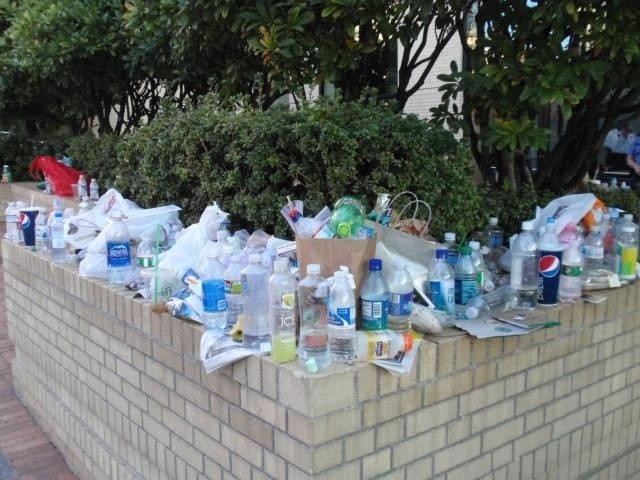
[295,234,377,329]
[364,216,434,270]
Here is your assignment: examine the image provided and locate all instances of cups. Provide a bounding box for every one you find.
[18,194,37,248]
[70,183,81,201]
[142,264,175,313]
[314,206,332,227]
[279,199,304,233]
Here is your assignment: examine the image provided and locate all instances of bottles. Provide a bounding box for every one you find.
[613,212,639,281]
[200,231,298,366]
[46,153,71,193]
[364,219,376,240]
[1,164,12,182]
[89,177,100,201]
[615,226,637,283]
[327,269,359,367]
[78,174,88,197]
[37,201,92,262]
[290,211,336,240]
[299,263,332,374]
[332,194,366,239]
[106,212,158,298]
[354,328,412,362]
[389,207,623,333]
[360,258,386,332]
[5,201,17,241]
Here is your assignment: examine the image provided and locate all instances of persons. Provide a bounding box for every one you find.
[626,136,640,184]
[601,123,636,171]
